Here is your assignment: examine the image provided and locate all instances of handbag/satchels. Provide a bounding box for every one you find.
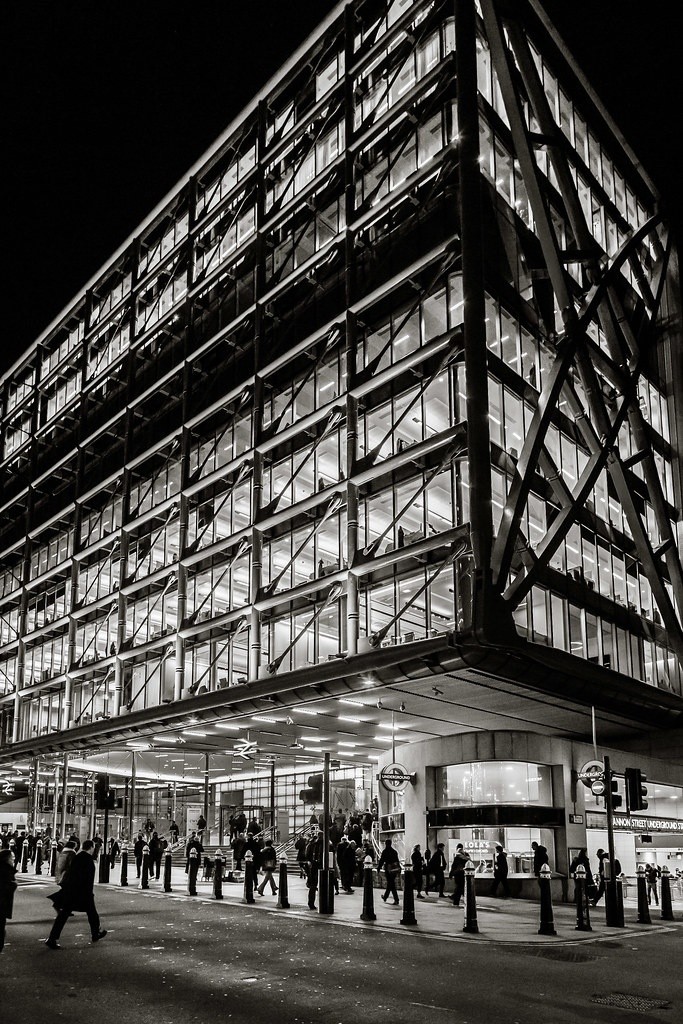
[264,859,274,869]
[388,862,401,874]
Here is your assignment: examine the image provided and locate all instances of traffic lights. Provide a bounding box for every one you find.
[611,780,622,810]
[94,776,123,810]
[628,769,648,810]
[299,774,323,804]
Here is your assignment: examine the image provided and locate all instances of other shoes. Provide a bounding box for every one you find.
[45,937,60,948]
[149,876,155,880]
[425,887,429,895]
[136,877,140,878]
[588,896,597,906]
[381,894,386,902]
[393,901,399,906]
[335,891,339,895]
[417,894,426,899]
[254,888,258,891]
[448,894,454,899]
[92,929,108,942]
[438,894,447,898]
[349,888,355,893]
[258,890,264,896]
[273,892,277,895]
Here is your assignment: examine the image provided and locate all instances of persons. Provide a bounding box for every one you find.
[490,846,508,898]
[532,842,549,878]
[328,808,374,892]
[92,833,121,869]
[170,821,179,843]
[197,815,206,834]
[231,832,279,896]
[645,863,660,906]
[0,823,80,865]
[229,814,261,844]
[377,839,401,904]
[295,831,323,909]
[185,836,204,874]
[570,848,609,906]
[134,818,164,881]
[411,844,425,899]
[448,843,469,906]
[310,811,332,831]
[0,849,18,951]
[45,841,107,949]
[425,843,448,898]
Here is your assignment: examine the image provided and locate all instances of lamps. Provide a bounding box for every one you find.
[667,853,681,860]
[377,699,382,709]
[399,703,406,713]
[640,831,654,844]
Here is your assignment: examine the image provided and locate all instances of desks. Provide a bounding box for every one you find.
[623,882,631,897]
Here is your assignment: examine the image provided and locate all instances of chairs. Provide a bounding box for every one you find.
[219,678,228,688]
[404,631,415,642]
[325,654,336,661]
[237,677,247,683]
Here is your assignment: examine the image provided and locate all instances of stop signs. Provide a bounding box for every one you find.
[592,782,604,794]
